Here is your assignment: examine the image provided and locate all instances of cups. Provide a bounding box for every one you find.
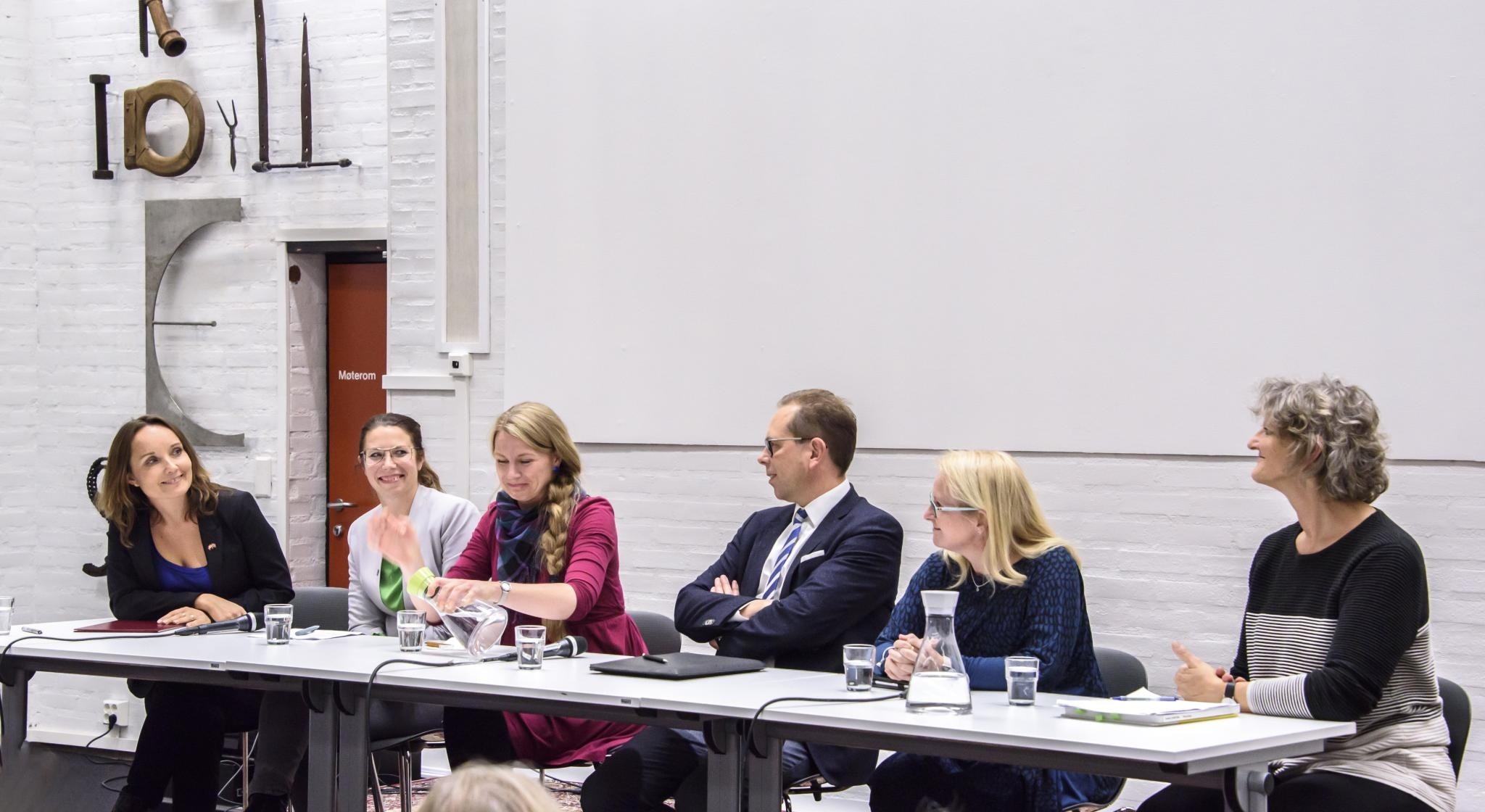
[514,625,547,670]
[397,609,427,652]
[1005,656,1041,705]
[0,596,15,635]
[263,604,294,645]
[842,644,876,690]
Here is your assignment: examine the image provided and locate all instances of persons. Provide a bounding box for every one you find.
[366,402,650,771]
[869,450,1122,812]
[244,412,483,812]
[417,759,562,812]
[1135,376,1459,811]
[95,415,295,811]
[580,388,904,812]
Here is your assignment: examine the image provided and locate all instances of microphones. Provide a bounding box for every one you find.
[173,613,266,636]
[498,635,588,662]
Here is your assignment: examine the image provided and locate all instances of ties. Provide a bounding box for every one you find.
[762,510,808,599]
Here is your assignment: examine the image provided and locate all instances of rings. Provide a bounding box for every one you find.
[910,646,916,651]
[190,615,196,620]
[180,612,186,616]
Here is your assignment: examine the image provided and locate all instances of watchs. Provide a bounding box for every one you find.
[1220,681,1238,705]
[494,580,511,606]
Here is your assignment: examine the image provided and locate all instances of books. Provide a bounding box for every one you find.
[1056,685,1240,727]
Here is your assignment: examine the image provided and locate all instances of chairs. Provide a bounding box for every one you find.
[238,589,1472,812]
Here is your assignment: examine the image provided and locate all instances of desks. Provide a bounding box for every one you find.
[0,617,1360,812]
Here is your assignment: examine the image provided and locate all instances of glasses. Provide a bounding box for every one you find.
[930,492,979,518]
[360,445,420,465]
[765,437,812,458]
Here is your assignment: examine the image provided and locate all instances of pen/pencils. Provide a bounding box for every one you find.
[1111,696,1180,702]
[643,653,669,663]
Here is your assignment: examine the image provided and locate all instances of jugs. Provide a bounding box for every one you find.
[407,567,509,658]
[904,590,973,716]
